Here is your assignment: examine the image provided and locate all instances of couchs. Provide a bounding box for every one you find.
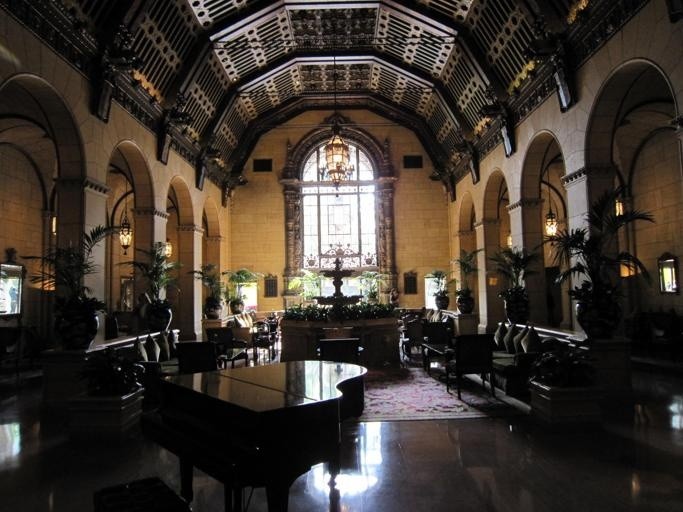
[227,321,253,347]
[482,334,555,394]
[118,342,217,404]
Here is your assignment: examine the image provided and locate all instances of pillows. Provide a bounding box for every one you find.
[131,330,181,363]
[493,320,540,355]
[234,313,254,327]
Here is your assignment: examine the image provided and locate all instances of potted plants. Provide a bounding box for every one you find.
[532,182,657,340]
[19,224,122,350]
[429,268,459,310]
[222,269,256,314]
[487,243,544,324]
[186,263,235,319]
[66,346,145,449]
[358,272,385,305]
[115,241,184,331]
[529,346,604,425]
[453,248,487,314]
[289,274,321,310]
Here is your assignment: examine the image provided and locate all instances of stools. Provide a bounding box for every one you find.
[92,475,193,512]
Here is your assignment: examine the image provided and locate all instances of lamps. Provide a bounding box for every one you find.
[615,199,624,219]
[119,178,132,256]
[324,24,350,190]
[166,238,173,258]
[544,167,558,238]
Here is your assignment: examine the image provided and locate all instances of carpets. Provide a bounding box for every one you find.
[348,367,527,421]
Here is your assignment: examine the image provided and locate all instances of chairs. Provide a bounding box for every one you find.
[205,327,248,368]
[319,338,360,364]
[400,312,494,400]
[252,321,275,361]
